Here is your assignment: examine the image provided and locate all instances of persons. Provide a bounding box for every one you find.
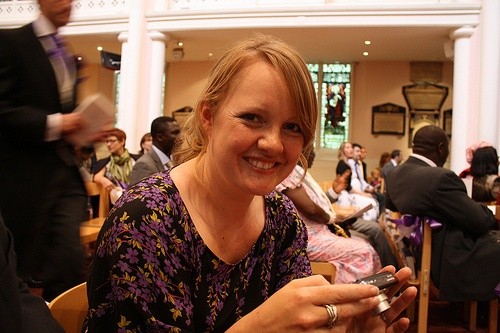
[85,105,194,211]
[386,125,500,301]
[273,143,382,285]
[80,34,417,333]
[470,146,500,203]
[372,149,403,181]
[336,142,386,221]
[0,0,84,333]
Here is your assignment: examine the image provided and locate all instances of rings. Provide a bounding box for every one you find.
[324,304,338,328]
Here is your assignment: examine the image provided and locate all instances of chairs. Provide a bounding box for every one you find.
[311,178,500,333]
[45,180,108,333]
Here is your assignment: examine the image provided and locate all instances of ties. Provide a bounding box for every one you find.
[355,163,363,187]
[166,160,173,169]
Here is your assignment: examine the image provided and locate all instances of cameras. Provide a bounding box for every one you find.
[353,272,397,316]
